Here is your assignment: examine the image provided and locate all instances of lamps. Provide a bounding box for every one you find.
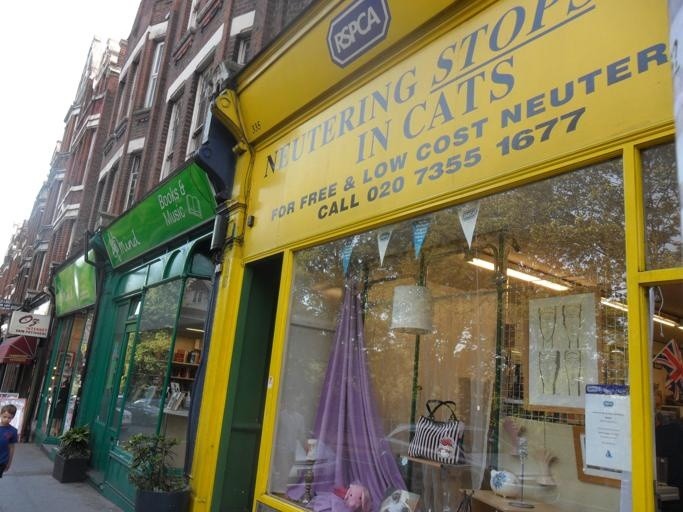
[294,434,328,504]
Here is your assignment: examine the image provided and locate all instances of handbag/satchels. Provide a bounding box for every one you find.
[408,400,466,465]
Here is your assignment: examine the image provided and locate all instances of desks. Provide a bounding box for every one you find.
[399,454,472,512]
[458,488,563,512]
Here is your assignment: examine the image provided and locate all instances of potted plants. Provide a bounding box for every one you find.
[122,431,192,512]
[51,427,92,484]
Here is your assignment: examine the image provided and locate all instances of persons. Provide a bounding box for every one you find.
[54,375,70,436]
[0,404,17,478]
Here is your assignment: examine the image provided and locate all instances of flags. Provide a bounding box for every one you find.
[654,339,683,391]
